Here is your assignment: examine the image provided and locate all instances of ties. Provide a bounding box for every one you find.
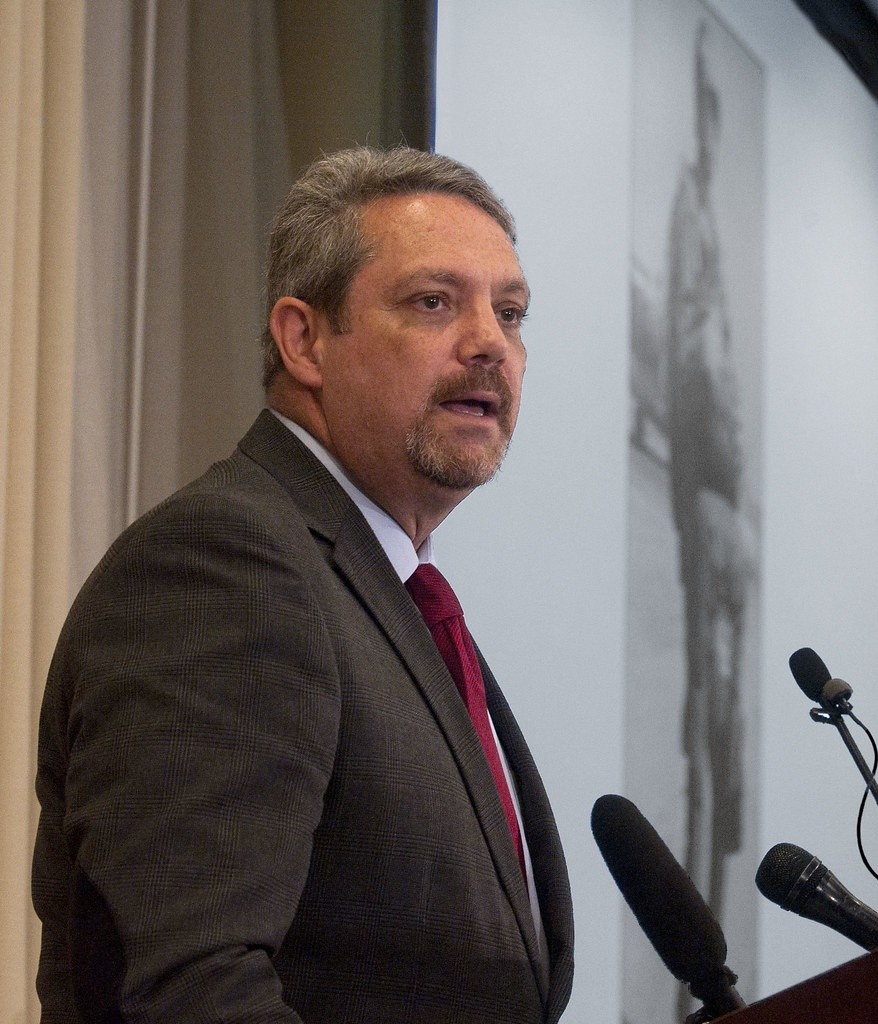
[406,565,530,902]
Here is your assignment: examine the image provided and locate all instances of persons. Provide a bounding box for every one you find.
[30,145,575,1024]
[665,23,752,1024]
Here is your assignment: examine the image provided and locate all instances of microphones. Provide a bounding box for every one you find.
[591,795,749,1018]
[822,678,852,704]
[789,647,878,806]
[755,842,878,951]
